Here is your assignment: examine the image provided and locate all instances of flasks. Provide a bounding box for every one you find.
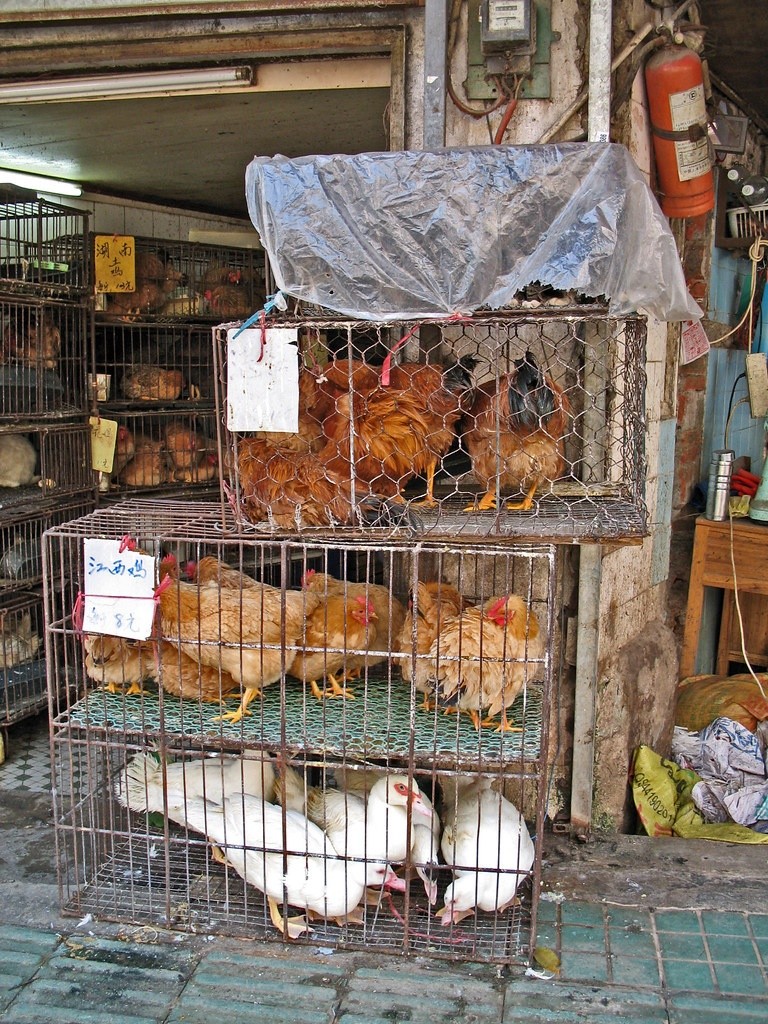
[704,449,735,523]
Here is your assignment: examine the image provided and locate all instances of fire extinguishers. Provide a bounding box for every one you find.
[634,1,716,219]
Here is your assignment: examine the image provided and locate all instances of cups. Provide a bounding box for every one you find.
[100,471,109,492]
[91,285,108,311]
[87,373,111,401]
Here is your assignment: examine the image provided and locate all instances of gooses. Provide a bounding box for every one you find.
[116,730,536,938]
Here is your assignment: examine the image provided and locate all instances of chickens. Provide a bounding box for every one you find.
[0,250,567,734]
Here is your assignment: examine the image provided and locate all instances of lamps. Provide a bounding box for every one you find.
[0,62,258,108]
[0,167,85,197]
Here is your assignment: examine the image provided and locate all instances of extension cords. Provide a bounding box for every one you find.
[745,352,768,419]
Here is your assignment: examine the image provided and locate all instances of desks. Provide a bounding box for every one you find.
[679,514,768,684]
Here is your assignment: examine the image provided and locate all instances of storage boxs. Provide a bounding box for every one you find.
[0,197,650,968]
[725,204,768,260]
